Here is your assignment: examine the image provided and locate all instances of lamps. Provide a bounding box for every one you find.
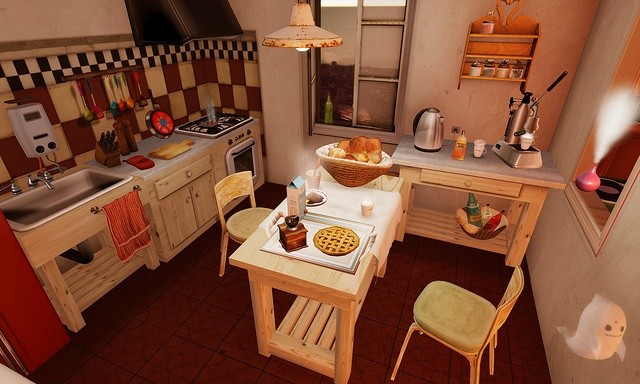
[261,0,343,52]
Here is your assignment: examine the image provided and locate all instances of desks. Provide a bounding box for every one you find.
[12,176,160,333]
[228,164,404,384]
[391,135,567,268]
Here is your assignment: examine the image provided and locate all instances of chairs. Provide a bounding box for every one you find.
[391,265,525,384]
[214,171,274,277]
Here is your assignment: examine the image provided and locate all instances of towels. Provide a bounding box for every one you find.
[101,190,153,264]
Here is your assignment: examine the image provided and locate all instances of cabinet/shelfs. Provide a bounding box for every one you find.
[144,143,225,263]
[458,0,541,94]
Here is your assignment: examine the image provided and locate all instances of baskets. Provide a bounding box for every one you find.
[316,142,393,187]
[456,206,508,239]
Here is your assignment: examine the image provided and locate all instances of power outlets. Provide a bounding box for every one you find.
[452,126,460,133]
